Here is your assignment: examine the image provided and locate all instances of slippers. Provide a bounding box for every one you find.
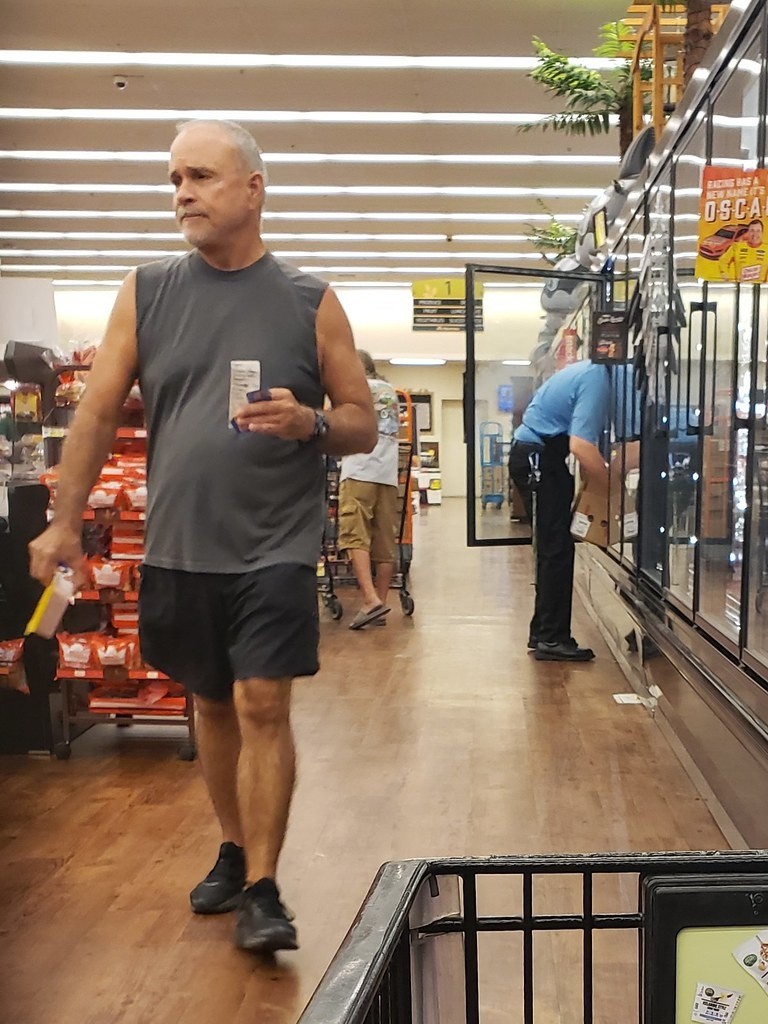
[348,607,391,629]
[371,621,386,626]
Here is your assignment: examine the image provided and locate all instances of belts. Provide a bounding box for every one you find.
[515,444,548,456]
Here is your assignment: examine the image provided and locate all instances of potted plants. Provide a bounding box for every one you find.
[512,21,644,160]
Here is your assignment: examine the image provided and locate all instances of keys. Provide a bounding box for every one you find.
[527,470,541,485]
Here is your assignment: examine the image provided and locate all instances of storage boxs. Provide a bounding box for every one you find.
[483,466,509,480]
[482,481,508,494]
[569,471,610,548]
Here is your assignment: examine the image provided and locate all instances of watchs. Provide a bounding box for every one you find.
[310,409,329,443]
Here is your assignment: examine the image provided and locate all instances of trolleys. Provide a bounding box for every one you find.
[318,443,414,619]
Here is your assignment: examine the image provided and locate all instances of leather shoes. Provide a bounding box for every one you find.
[535,641,595,660]
[527,635,579,649]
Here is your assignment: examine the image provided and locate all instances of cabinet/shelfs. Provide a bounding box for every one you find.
[0,335,420,767]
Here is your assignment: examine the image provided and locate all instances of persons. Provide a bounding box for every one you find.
[28,120,379,952]
[337,349,399,629]
[508,358,611,662]
[612,363,669,653]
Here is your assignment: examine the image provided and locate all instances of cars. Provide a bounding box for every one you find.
[699,225,748,260]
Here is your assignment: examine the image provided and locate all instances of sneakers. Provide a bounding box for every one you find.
[233,876,299,953]
[190,842,244,913]
